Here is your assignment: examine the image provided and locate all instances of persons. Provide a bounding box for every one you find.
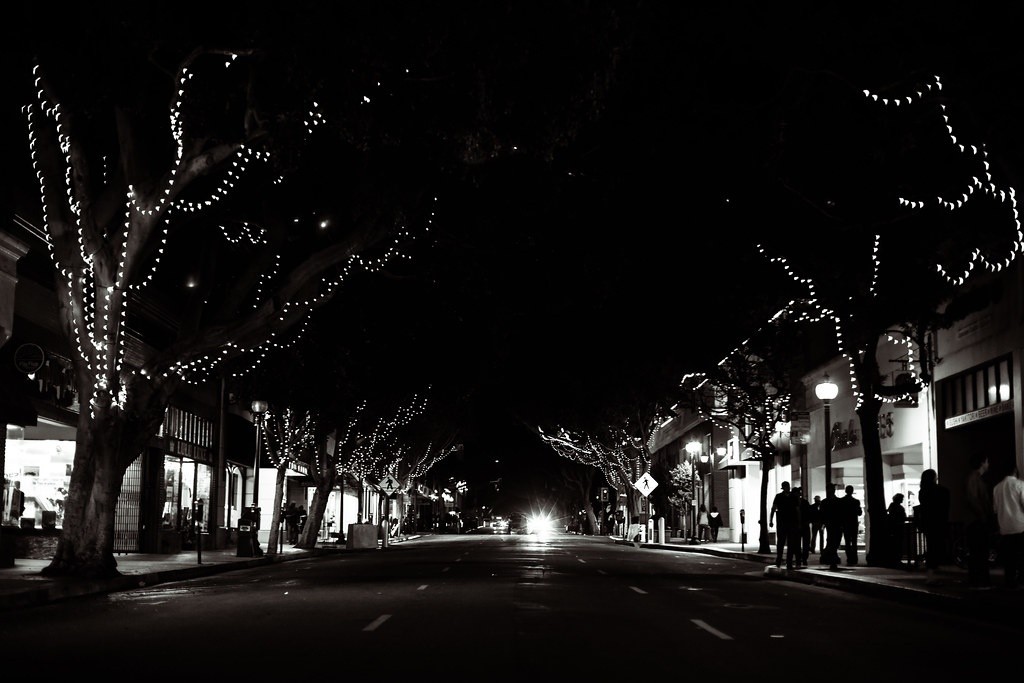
[991,460,1024,587]
[767,481,861,571]
[947,456,989,583]
[708,505,723,540]
[968,452,992,495]
[888,494,911,572]
[697,503,710,540]
[279,497,308,543]
[912,469,964,581]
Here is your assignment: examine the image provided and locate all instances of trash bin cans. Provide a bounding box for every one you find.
[237,519,253,556]
